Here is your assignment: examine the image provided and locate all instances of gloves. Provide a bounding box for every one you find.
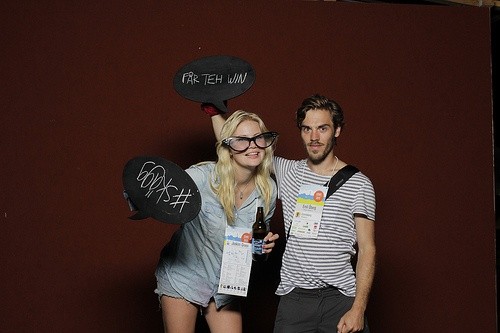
[201,102,222,116]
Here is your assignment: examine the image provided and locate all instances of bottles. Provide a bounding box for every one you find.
[252,207,268,262]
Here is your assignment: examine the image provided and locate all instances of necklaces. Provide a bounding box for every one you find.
[237,175,253,199]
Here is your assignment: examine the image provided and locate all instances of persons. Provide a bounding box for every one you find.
[201,94,377,333]
[122,110,280,333]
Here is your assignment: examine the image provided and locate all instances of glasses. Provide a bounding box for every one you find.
[222,131,279,153]
